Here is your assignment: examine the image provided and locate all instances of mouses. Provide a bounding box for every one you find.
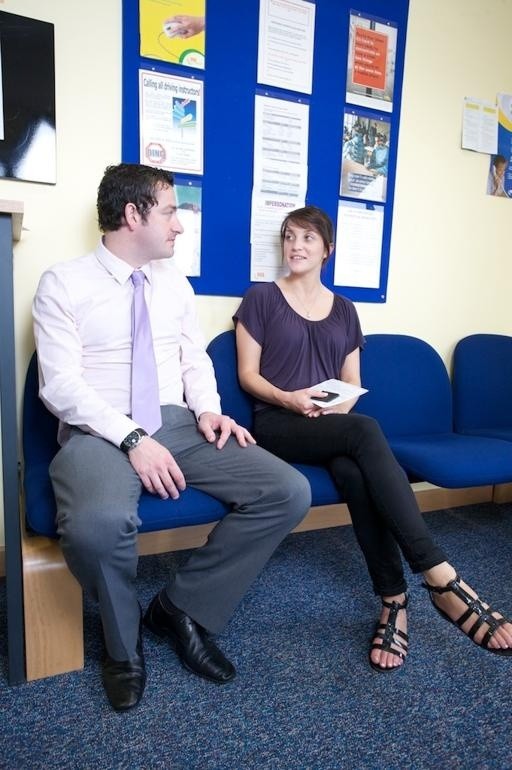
[163,20,186,36]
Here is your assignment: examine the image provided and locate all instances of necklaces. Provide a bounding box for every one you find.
[289,282,323,319]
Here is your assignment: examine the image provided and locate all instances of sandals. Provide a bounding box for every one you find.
[421,574,512,656]
[368,593,412,674]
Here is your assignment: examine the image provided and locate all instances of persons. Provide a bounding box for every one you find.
[488,156,508,196]
[343,119,388,177]
[31,164,314,713]
[232,207,512,674]
[165,13,205,40]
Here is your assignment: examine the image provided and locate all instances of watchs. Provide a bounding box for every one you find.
[119,425,148,454]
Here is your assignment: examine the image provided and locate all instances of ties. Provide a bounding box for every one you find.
[131,270,162,437]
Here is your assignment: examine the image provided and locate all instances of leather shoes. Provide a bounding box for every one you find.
[142,591,237,683]
[102,644,147,711]
